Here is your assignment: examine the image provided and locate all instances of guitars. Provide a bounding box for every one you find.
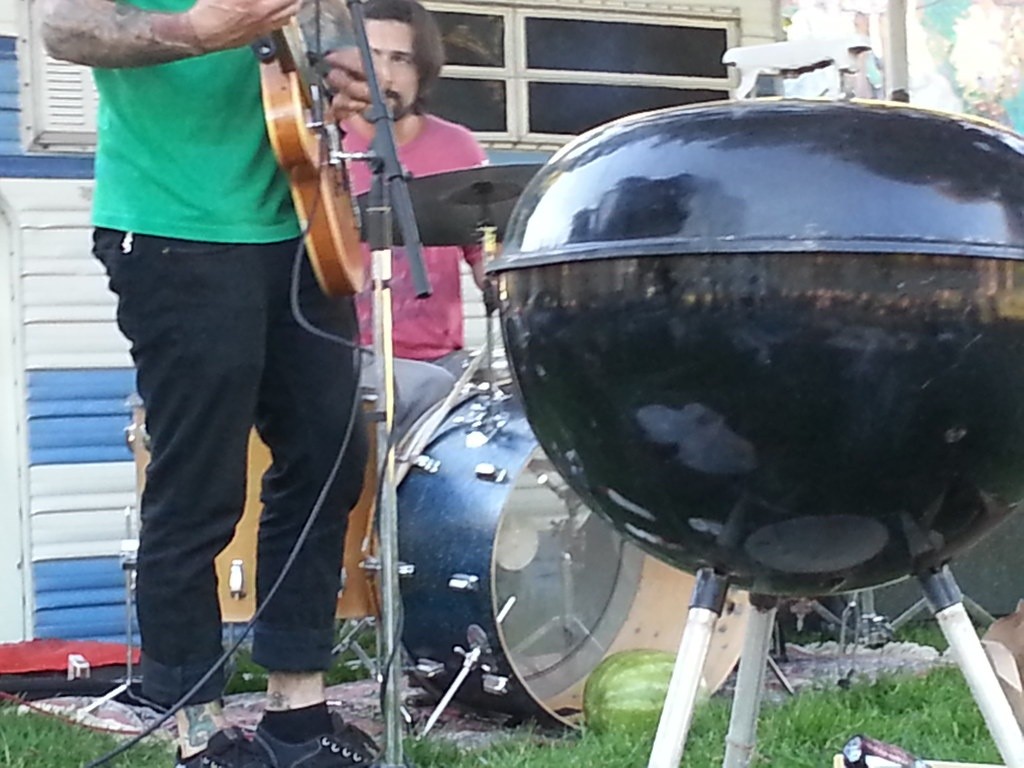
[250,2,366,301]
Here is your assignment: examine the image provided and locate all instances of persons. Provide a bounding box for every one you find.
[41,1,387,767]
[337,0,504,443]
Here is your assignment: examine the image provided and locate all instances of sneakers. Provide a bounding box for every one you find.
[173,726,272,768]
[254,709,384,768]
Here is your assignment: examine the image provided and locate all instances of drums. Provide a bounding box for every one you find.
[123,384,372,631]
[367,375,757,735]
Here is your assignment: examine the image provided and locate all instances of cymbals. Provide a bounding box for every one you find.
[356,162,546,244]
